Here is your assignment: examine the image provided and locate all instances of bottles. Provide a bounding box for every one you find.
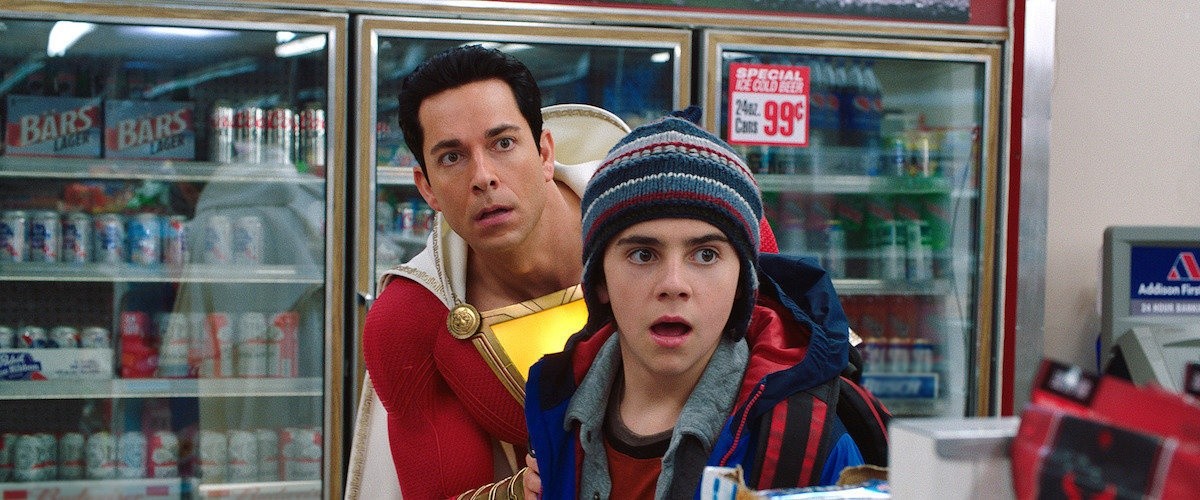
[728,57,883,176]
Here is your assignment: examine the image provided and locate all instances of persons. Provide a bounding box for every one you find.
[524,106,893,500]
[155,115,406,500]
[345,45,779,500]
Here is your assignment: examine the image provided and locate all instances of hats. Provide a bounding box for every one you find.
[582,106,764,344]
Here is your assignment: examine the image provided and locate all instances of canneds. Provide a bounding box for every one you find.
[206,99,324,168]
[0,426,321,483]
[835,295,933,374]
[378,99,435,241]
[0,209,262,266]
[116,310,302,376]
[761,193,934,282]
[0,324,112,351]
[742,129,941,178]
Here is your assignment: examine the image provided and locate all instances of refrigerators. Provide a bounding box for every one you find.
[0,0,1025,500]
